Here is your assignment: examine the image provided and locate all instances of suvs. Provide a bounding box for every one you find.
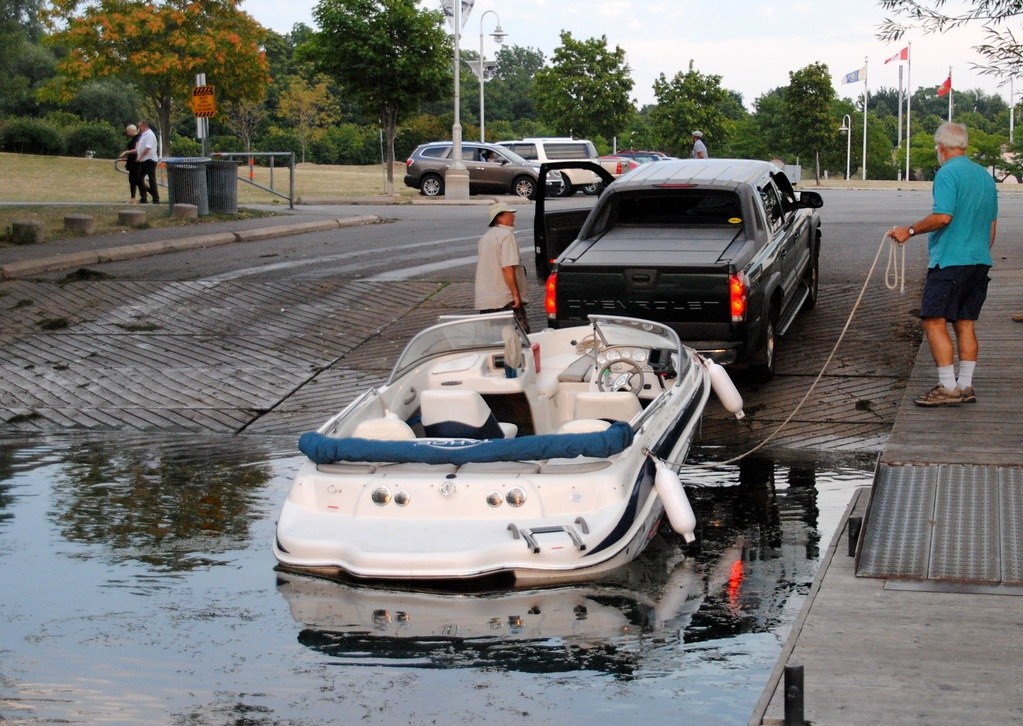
[404,141,563,201]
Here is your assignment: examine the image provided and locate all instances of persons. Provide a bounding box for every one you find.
[691,130,708,159]
[770,155,785,171]
[887,121,998,408]
[119,123,153,204]
[135,119,160,204]
[478,148,499,162]
[473,201,532,335]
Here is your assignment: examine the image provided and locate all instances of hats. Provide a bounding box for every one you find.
[692,131,704,138]
[487,201,518,228]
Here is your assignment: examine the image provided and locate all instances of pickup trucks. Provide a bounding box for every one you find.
[582,161,631,195]
[534,160,825,386]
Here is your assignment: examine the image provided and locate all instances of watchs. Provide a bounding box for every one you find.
[908,226,916,237]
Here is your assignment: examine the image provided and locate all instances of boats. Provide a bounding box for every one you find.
[272,309,712,592]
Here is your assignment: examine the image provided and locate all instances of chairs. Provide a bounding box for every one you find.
[421,390,517,440]
[574,392,643,423]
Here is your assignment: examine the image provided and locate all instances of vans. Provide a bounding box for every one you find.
[495,137,602,198]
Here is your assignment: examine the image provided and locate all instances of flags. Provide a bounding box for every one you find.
[884,47,908,64]
[936,77,951,96]
[842,66,866,86]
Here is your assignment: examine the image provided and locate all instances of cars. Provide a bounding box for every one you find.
[599,151,677,171]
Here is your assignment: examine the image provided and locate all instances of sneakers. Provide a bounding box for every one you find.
[960,385,977,403]
[912,380,964,407]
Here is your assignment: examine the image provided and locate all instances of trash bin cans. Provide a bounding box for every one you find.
[162,156,212,215]
[206,161,243,214]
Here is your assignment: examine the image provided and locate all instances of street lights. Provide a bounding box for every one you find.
[479,10,509,143]
[839,114,851,180]
